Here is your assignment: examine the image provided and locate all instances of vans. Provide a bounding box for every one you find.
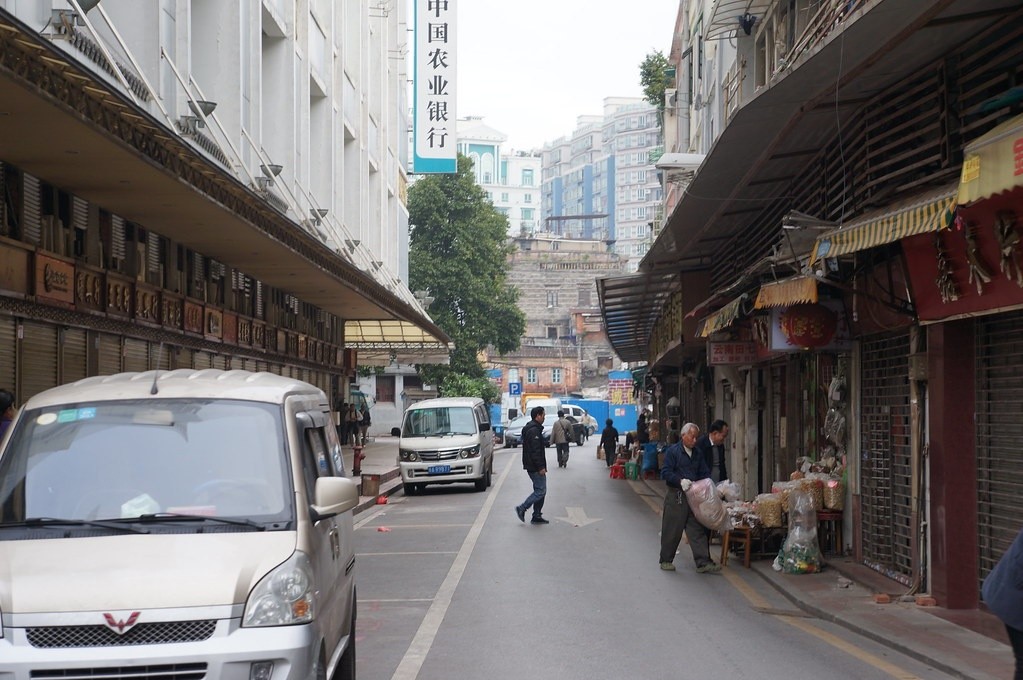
[0,365,358,680]
[525,398,563,414]
[391,397,492,494]
[562,404,598,436]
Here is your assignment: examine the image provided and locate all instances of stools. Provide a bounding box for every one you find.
[610,465,626,480]
[615,458,628,465]
[721,526,753,568]
[625,462,640,481]
[643,470,655,480]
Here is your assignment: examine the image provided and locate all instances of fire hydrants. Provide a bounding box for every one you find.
[350,444,366,475]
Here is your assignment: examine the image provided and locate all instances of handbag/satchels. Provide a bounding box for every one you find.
[564,431,573,442]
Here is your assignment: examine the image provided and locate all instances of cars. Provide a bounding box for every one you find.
[504,415,585,448]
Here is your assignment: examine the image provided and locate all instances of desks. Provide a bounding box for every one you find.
[729,508,843,557]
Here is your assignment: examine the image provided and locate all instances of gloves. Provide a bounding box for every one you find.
[681,478,692,491]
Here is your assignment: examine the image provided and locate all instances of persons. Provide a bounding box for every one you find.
[983,529,1023,680]
[339,403,349,446]
[659,423,722,574]
[345,404,363,446]
[581,410,589,441]
[358,403,370,447]
[514,406,548,524]
[694,420,729,484]
[637,409,651,448]
[626,430,637,449]
[600,419,618,468]
[549,410,574,468]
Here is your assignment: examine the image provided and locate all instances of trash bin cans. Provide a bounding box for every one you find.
[492,425,503,444]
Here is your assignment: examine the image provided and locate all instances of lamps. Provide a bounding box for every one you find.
[254,165,284,191]
[180,100,217,132]
[51,0,100,30]
[339,239,361,255]
[308,208,329,228]
[389,279,401,290]
[367,261,383,275]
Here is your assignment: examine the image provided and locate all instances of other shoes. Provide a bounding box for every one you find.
[659,562,676,570]
[516,506,525,522]
[531,517,549,524]
[696,563,715,573]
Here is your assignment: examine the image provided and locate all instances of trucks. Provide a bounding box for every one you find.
[521,393,551,414]
[351,391,376,413]
